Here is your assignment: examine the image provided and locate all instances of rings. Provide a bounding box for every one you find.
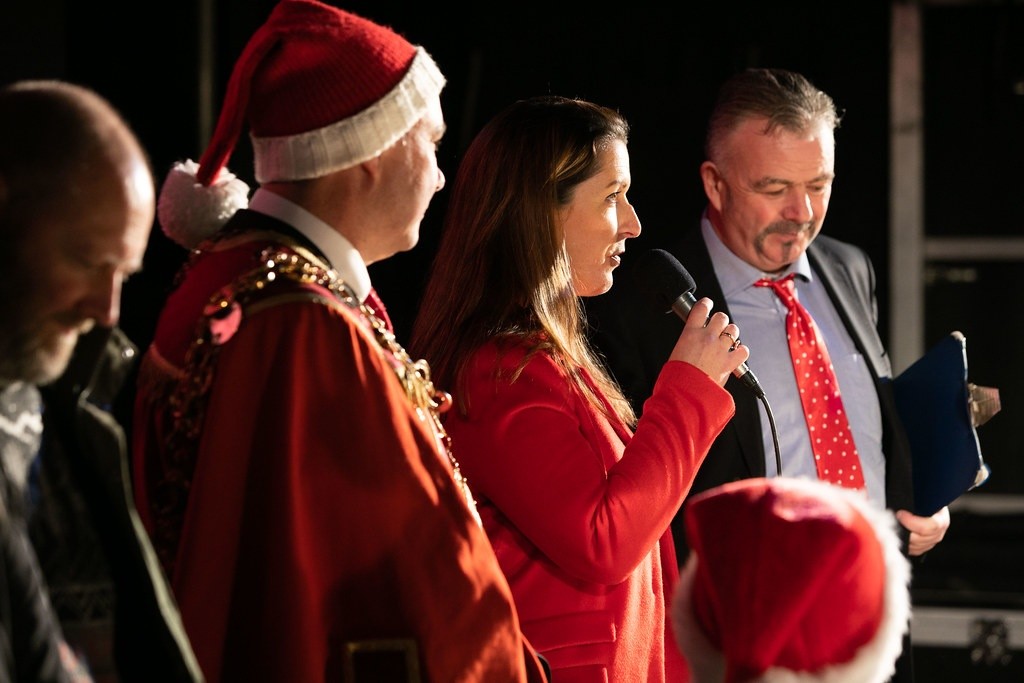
[721,331,738,348]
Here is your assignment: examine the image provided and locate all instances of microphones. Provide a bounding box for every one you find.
[632,248,766,398]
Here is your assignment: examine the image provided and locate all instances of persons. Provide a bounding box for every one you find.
[673,475,913,683]
[0,81,207,683]
[406,97,744,683]
[128,0,552,683]
[583,68,949,683]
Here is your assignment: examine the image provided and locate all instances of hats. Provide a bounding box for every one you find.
[156,2,446,244]
[683,472,911,683]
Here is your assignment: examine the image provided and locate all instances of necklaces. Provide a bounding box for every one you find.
[162,251,480,504]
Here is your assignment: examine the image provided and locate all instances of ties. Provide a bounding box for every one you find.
[750,273,871,490]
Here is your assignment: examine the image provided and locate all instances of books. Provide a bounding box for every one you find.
[894,331,989,518]
